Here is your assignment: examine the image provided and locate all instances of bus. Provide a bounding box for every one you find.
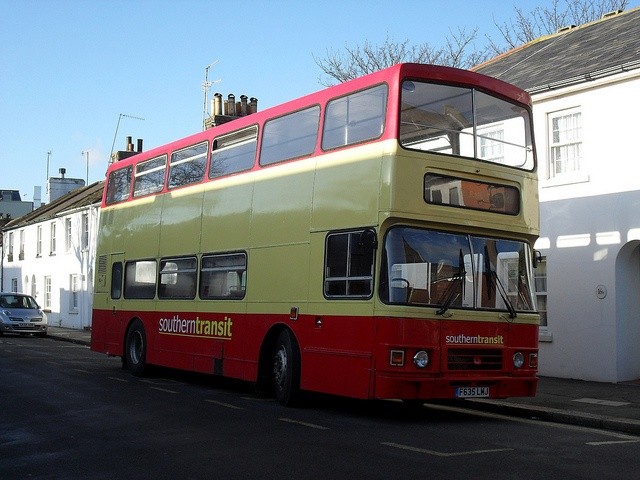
[89,60,540,407]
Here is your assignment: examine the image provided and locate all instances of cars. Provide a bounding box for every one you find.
[1,292,48,338]
